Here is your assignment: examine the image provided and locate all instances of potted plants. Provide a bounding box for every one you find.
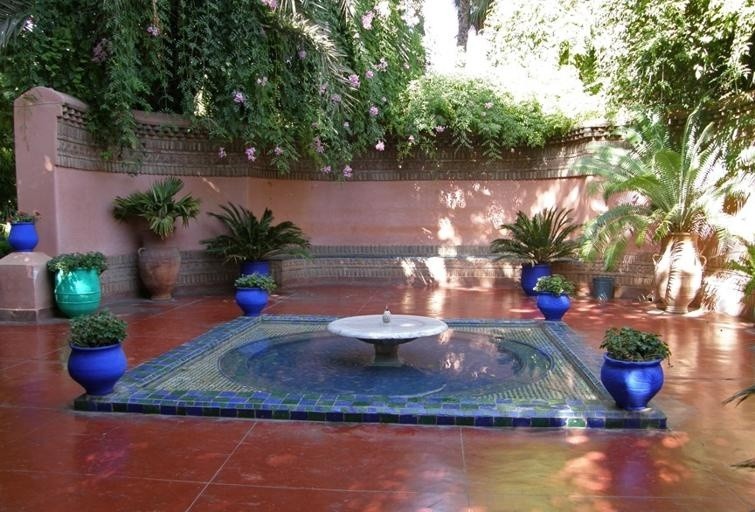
[199,201,314,278]
[491,207,586,297]
[114,176,200,299]
[574,103,728,315]
[533,274,576,322]
[46,251,108,318]
[67,308,128,396]
[234,272,277,317]
[599,328,672,411]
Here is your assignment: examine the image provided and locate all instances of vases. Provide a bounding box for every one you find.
[9,222,39,252]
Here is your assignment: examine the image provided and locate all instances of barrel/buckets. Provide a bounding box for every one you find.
[593,277,613,300]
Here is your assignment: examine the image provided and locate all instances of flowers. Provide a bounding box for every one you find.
[7,208,44,222]
